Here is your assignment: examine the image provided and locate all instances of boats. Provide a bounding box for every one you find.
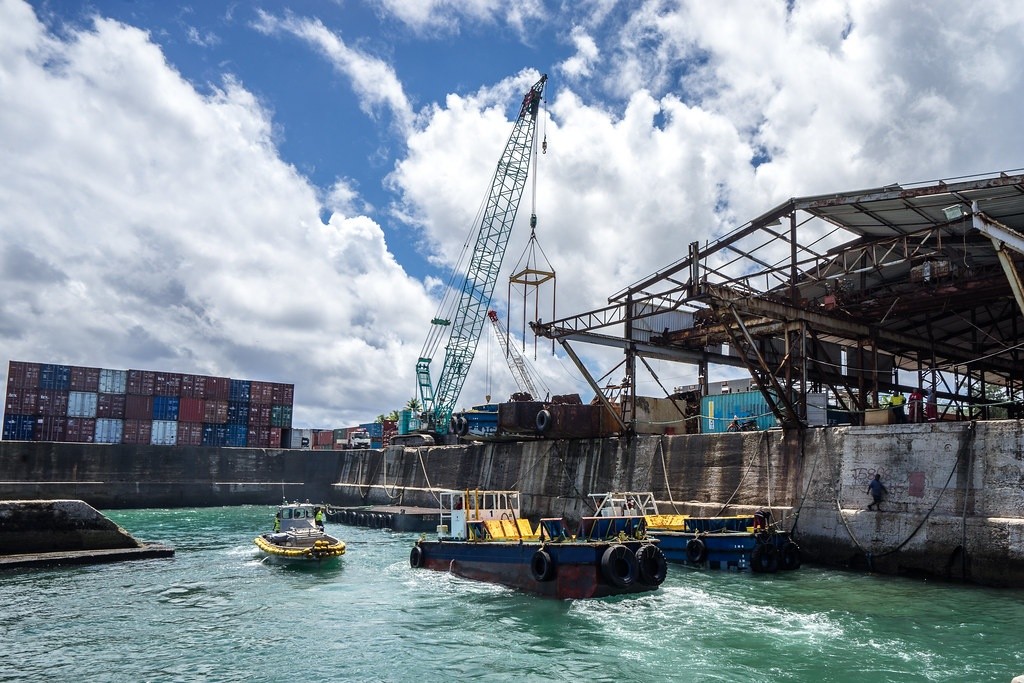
[255,499,346,560]
[536,492,801,576]
[410,488,668,599]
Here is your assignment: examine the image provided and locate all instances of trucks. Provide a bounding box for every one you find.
[337,431,371,449]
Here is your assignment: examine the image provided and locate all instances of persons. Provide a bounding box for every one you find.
[623,499,635,516]
[303,511,309,518]
[926,389,935,421]
[295,512,300,519]
[908,389,922,423]
[890,390,906,423]
[315,507,325,532]
[596,498,605,516]
[455,497,471,510]
[273,513,281,533]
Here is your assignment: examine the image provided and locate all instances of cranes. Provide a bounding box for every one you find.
[389,73,557,446]
[485,308,622,439]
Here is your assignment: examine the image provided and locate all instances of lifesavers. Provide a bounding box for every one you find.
[635,542,670,588]
[684,539,707,564]
[536,410,552,433]
[777,541,805,572]
[600,545,640,588]
[530,548,552,583]
[410,544,425,571]
[749,541,777,576]
